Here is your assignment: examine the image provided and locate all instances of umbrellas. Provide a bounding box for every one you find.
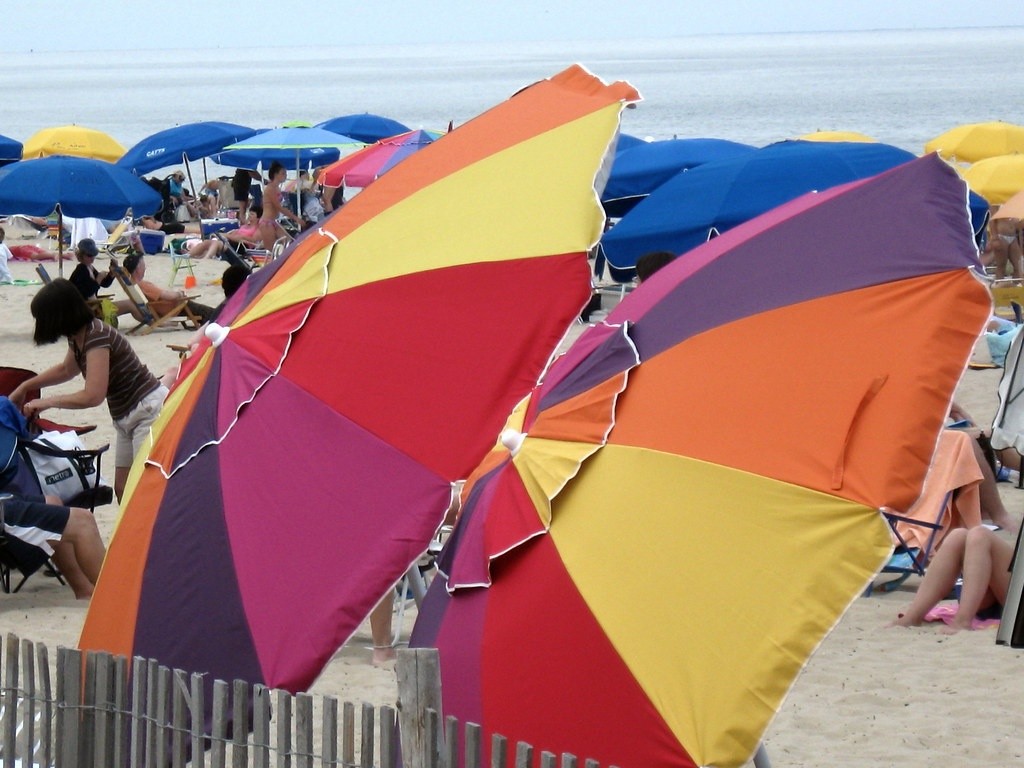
[0,112,450,276]
[76,63,639,768]
[405,156,994,768]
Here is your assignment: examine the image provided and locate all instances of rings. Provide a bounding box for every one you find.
[26,403,30,406]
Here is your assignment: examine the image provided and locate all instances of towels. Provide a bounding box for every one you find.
[71,217,110,251]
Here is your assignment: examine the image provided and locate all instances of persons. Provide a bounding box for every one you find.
[883,401,1024,636]
[231,169,269,225]
[198,177,225,218]
[983,231,993,266]
[989,218,1022,283]
[295,170,312,193]
[162,265,252,387]
[141,216,202,235]
[636,252,677,285]
[311,165,337,216]
[0,494,106,599]
[601,121,1024,284]
[186,205,263,259]
[67,239,145,322]
[0,228,13,283]
[9,244,73,263]
[123,251,214,322]
[7,277,170,506]
[370,588,399,671]
[170,171,200,217]
[260,162,306,251]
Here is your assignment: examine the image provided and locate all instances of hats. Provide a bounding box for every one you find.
[78,238,100,256]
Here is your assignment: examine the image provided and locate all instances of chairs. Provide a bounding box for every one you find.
[857,277,1024,598]
[0,181,301,594]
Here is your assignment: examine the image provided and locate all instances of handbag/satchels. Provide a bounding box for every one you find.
[24,429,104,503]
